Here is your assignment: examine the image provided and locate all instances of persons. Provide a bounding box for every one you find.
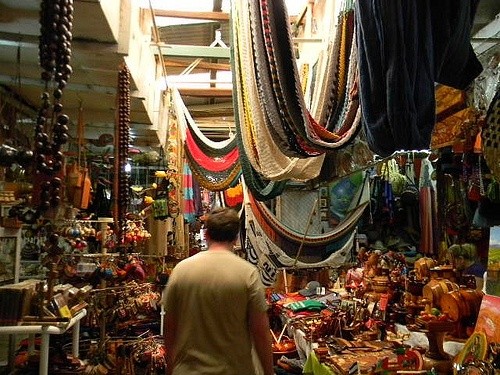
[163,208,275,375]
[448,244,486,278]
